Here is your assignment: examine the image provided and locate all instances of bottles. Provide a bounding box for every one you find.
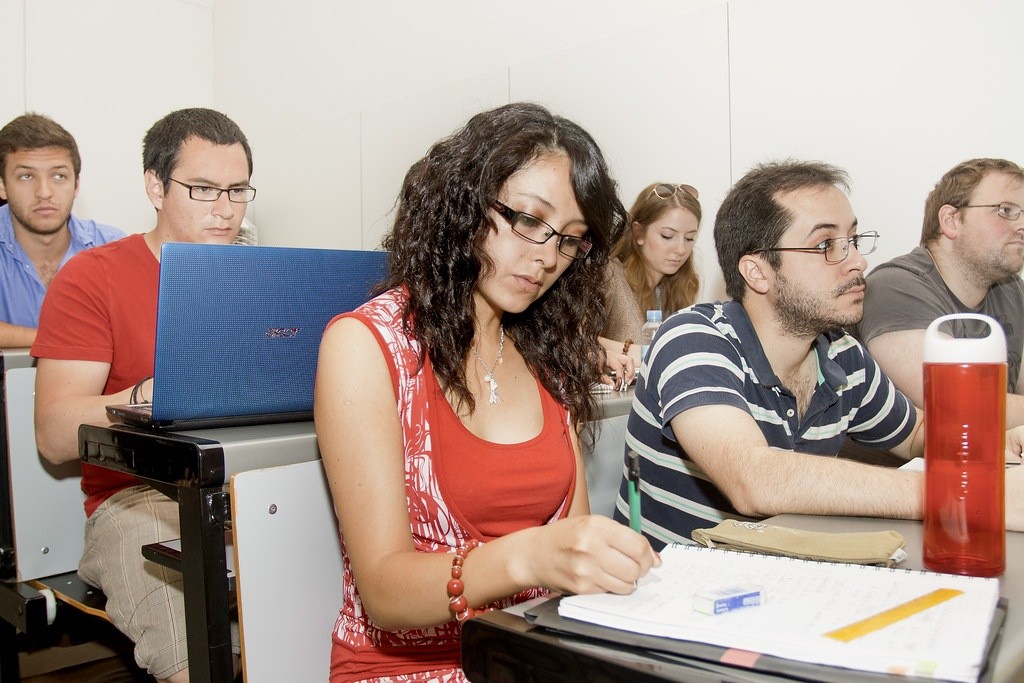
[642,310,663,360]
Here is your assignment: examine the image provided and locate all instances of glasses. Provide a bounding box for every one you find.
[750,230,880,265]
[645,182,699,202]
[490,198,593,261]
[164,176,258,204]
[953,202,1024,221]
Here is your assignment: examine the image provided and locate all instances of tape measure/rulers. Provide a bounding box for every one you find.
[823,587,964,643]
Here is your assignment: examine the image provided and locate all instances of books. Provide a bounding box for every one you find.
[558,543,1000,683]
[894,457,1009,474]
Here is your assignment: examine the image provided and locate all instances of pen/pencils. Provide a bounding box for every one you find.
[626,454,642,533]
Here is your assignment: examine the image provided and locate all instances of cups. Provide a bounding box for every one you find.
[923,313,1008,579]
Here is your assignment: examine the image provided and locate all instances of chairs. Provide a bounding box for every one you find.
[227,456,344,683]
[4,367,128,637]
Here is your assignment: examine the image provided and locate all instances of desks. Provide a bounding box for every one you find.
[458,446,1024,681]
[75,382,634,683]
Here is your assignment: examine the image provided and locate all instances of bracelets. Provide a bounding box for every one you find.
[621,338,634,368]
[129,376,152,405]
[447,539,499,626]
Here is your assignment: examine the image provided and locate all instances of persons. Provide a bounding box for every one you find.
[610,163,1024,554]
[572,181,702,368]
[499,316,636,391]
[845,157,1024,432]
[30,107,257,683]
[313,103,662,683]
[0,114,127,347]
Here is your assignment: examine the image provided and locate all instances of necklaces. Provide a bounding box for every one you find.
[470,324,505,405]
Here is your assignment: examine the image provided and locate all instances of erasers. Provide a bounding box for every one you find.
[693,583,765,615]
